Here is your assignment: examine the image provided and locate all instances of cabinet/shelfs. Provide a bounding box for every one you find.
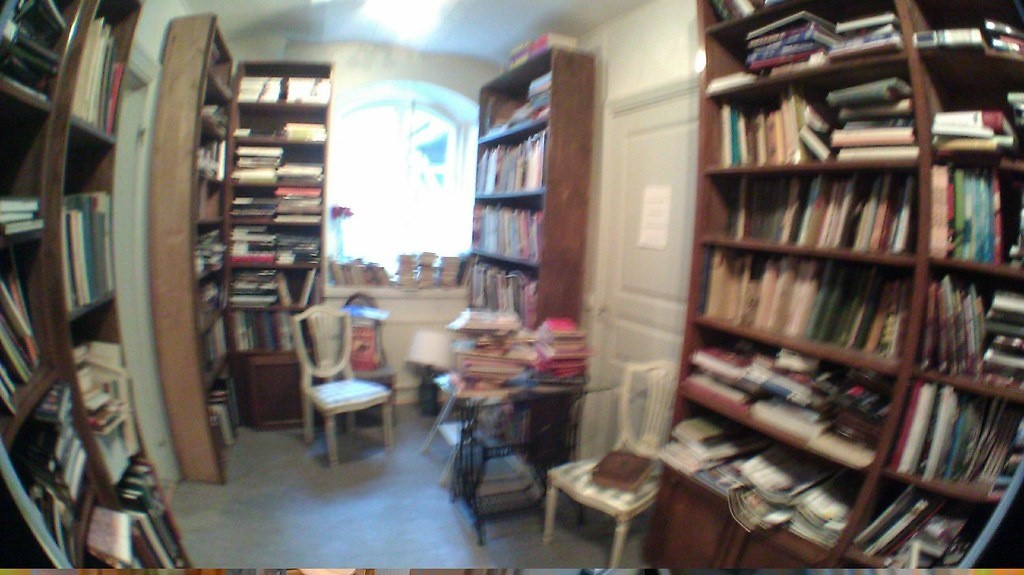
[0,0,193,569]
[223,56,333,358]
[149,14,238,486]
[643,0,1024,569]
[467,46,593,331]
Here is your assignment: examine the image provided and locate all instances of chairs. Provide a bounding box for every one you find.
[292,304,395,471]
[335,291,395,386]
[541,360,679,568]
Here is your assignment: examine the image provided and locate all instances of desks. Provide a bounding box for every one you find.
[433,367,618,545]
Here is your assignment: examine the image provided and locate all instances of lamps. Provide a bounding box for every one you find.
[403,333,453,416]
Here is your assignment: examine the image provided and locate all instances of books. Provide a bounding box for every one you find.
[0,1,182,572]
[664,1,1024,575]
[195,74,332,442]
[450,72,590,392]
